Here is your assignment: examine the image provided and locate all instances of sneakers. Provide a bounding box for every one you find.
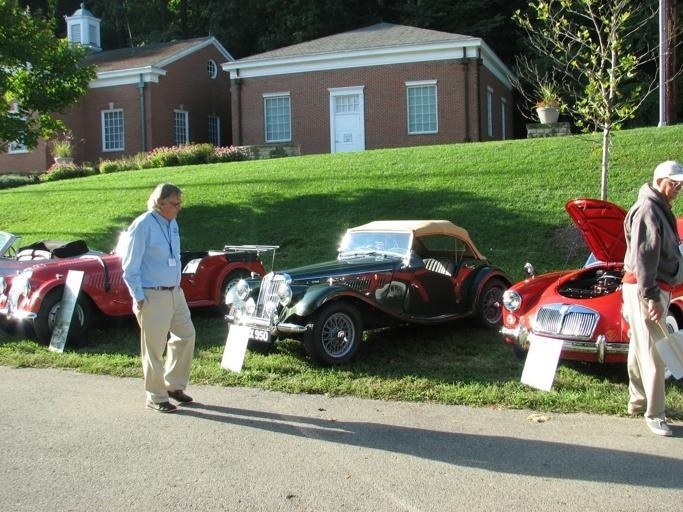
[646,417,673,436]
[628,403,645,414]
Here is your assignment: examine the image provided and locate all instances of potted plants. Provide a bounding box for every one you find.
[536,95,561,126]
[52,131,86,163]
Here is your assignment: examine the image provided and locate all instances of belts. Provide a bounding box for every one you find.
[142,285,175,291]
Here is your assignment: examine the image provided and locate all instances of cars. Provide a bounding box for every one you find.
[0,234,284,345]
[497,193,681,370]
[223,216,508,367]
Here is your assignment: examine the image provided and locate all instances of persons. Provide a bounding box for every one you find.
[122,183,196,412]
[621,160,683,435]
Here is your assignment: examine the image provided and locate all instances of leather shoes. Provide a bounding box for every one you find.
[146,402,177,413]
[168,391,193,402]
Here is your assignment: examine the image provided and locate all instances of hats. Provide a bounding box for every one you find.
[654,160,683,182]
